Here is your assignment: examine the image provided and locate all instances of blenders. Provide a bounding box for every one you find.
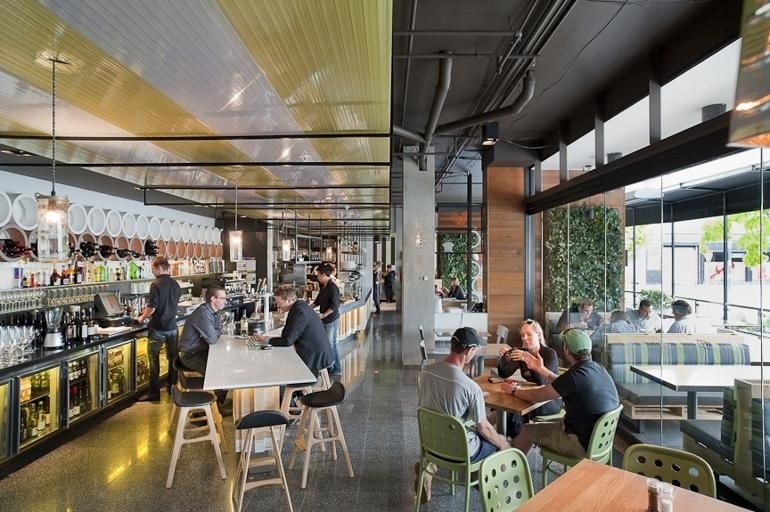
[43,307,66,348]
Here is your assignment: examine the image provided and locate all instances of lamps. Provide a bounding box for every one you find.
[36,61,72,265]
[725,0,770,152]
[282,206,291,263]
[481,122,498,147]
[229,175,243,262]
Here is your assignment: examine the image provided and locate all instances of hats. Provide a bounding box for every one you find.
[452,327,487,348]
[560,328,592,355]
[671,299,690,314]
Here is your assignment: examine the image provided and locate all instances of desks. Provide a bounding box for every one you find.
[202,332,317,453]
[471,371,558,438]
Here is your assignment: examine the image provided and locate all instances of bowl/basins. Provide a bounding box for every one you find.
[640,328,653,333]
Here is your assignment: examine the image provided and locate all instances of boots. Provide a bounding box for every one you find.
[375,308,380,314]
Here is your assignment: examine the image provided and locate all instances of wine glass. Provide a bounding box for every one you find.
[0,326,36,367]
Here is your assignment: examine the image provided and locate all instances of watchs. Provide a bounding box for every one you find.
[512,386,517,397]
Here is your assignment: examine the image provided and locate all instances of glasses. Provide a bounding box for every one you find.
[374,265,377,266]
[519,319,534,326]
[216,296,226,299]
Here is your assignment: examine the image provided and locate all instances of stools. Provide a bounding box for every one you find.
[166,356,198,430]
[287,380,355,489]
[315,361,335,390]
[230,409,294,512]
[177,371,228,455]
[165,383,228,488]
[279,383,327,452]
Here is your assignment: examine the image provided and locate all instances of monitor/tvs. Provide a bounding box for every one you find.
[95,290,124,317]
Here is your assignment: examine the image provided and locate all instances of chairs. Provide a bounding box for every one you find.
[419,324,426,338]
[496,324,508,343]
[413,406,496,512]
[419,339,433,370]
[477,447,534,512]
[622,443,717,500]
[540,405,624,488]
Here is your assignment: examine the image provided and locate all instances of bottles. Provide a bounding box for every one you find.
[241,310,248,331]
[21,401,47,441]
[71,384,89,405]
[0,312,45,350]
[63,310,94,343]
[646,477,659,512]
[68,359,86,381]
[12,262,138,287]
[123,296,145,317]
[658,483,673,512]
[171,258,206,275]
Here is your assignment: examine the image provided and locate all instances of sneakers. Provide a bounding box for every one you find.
[329,370,341,376]
[414,461,438,504]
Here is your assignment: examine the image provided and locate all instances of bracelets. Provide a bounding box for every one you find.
[324,314,327,318]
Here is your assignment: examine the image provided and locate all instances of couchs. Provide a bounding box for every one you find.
[605,333,750,422]
[545,310,612,367]
[679,377,770,495]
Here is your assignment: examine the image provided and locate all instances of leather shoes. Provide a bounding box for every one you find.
[165,383,172,394]
[135,392,160,402]
[286,410,302,429]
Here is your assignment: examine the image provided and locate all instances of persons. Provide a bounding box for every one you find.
[500,328,619,456]
[330,267,337,280]
[591,311,641,360]
[556,298,605,333]
[254,285,335,427]
[414,327,513,505]
[626,300,662,332]
[308,265,342,377]
[498,318,562,438]
[435,285,443,299]
[385,265,396,304]
[667,300,716,333]
[138,256,181,402]
[448,278,465,299]
[373,261,381,314]
[377,262,384,306]
[178,285,227,415]
